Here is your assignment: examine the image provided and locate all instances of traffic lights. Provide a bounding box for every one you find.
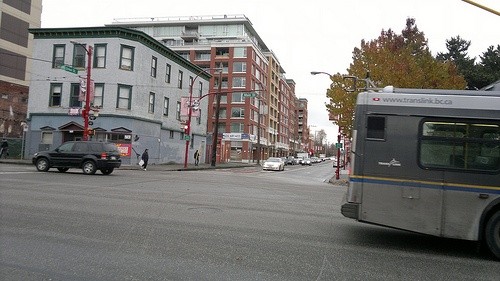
[184,124,189,134]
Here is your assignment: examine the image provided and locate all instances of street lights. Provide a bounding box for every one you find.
[183,68,223,167]
[70,41,92,141]
[311,71,342,179]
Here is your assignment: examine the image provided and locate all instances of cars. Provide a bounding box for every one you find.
[262,157,285,171]
[282,156,344,167]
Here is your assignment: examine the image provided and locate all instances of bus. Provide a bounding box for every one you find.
[339,70,500,258]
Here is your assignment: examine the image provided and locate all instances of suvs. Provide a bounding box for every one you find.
[32,141,121,175]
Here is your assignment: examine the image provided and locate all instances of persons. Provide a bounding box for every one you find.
[194,150,200,166]
[142,149,149,170]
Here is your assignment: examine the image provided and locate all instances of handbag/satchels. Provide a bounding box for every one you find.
[139,159,144,166]
